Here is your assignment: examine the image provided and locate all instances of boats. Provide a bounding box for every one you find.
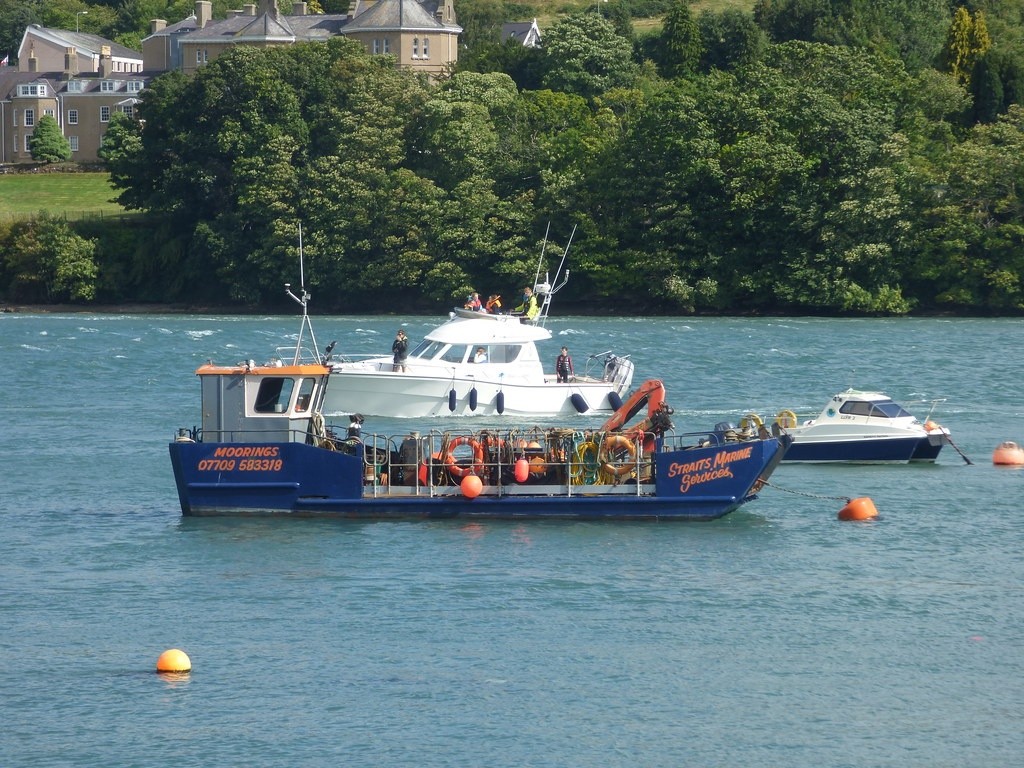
[275,219,635,419]
[167,357,795,521]
[715,388,952,465]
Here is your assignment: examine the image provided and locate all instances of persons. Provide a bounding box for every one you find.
[509,287,539,324]
[473,347,488,363]
[467,292,501,314]
[556,347,574,383]
[392,329,408,372]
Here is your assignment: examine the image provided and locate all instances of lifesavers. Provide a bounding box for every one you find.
[741,414,762,428]
[446,437,483,476]
[479,438,505,474]
[599,436,637,475]
[776,410,797,428]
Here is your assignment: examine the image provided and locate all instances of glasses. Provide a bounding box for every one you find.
[397,334,401,336]
